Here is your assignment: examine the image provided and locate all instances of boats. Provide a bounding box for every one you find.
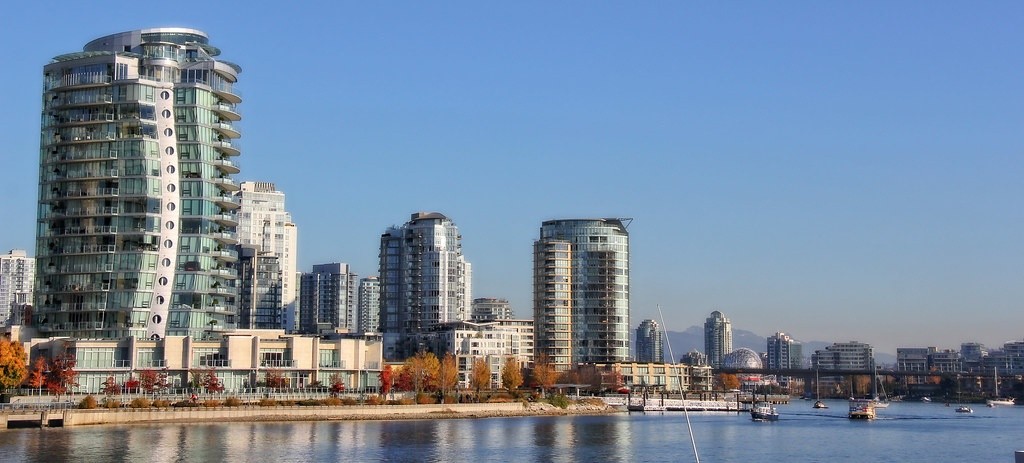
[956,405,973,414]
[812,401,829,408]
[985,396,1015,407]
[750,400,779,422]
[847,393,889,420]
